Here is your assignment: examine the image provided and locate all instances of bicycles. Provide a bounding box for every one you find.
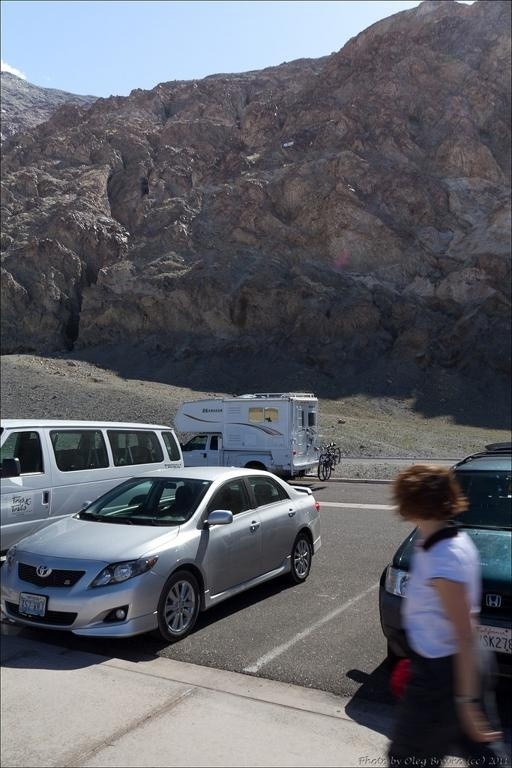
[316,441,341,482]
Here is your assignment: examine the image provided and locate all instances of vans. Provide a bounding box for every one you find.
[1,417,186,558]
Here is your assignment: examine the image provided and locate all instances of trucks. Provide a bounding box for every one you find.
[173,391,319,482]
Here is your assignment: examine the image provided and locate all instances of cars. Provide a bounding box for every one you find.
[0,465,322,644]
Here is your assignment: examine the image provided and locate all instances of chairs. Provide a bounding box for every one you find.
[253,484,274,504]
[171,486,195,517]
[122,444,151,464]
[14,439,42,473]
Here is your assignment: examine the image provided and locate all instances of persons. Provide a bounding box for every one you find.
[389,465,508,768]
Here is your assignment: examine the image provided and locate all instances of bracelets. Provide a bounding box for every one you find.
[453,695,481,704]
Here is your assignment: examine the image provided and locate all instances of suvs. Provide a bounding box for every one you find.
[378,441,512,689]
